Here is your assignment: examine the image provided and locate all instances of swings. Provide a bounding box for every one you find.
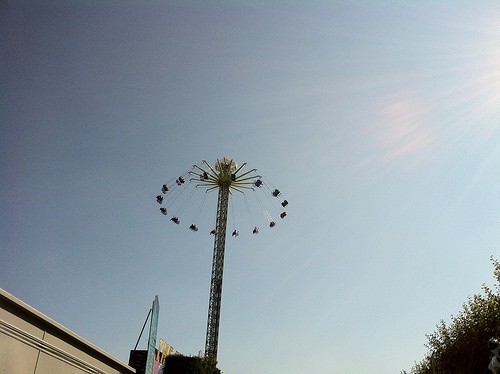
[157,159,288,237]
